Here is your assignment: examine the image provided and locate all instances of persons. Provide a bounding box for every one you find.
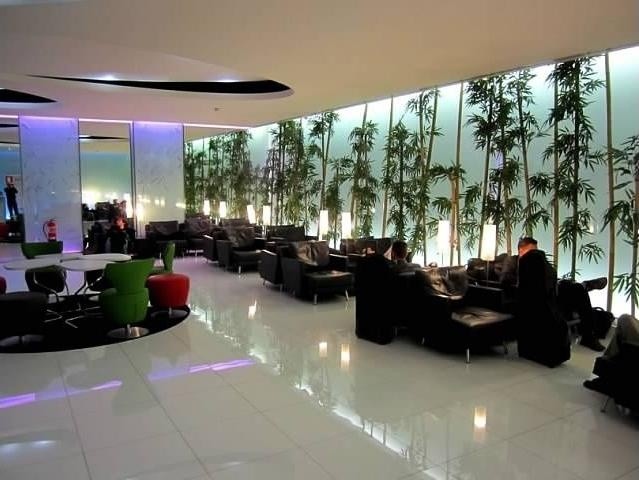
[501,237,608,352]
[110,216,136,256]
[582,314,639,393]
[3,181,20,220]
[387,240,422,273]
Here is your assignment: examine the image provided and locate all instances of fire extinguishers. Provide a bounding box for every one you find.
[43,217,57,242]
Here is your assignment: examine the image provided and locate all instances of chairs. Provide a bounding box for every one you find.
[0,241,190,350]
[356,259,582,364]
[89,212,358,305]
[585,346,638,417]
